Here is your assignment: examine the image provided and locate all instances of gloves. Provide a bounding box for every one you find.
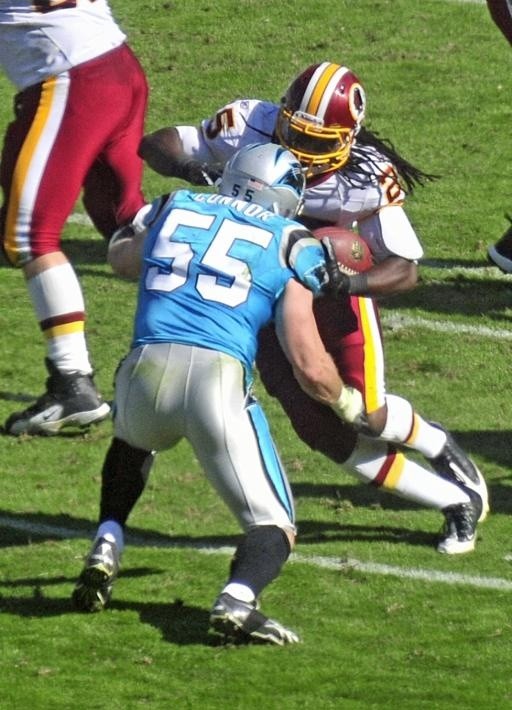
[314,234,351,299]
[181,157,225,187]
[341,384,369,433]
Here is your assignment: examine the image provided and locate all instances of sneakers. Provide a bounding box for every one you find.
[69,535,117,614]
[485,223,512,273]
[421,418,491,521]
[205,591,301,649]
[5,354,112,436]
[431,483,485,556]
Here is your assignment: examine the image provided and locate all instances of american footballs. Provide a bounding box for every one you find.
[312,225,372,279]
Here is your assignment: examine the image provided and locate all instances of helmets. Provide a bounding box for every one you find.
[282,61,367,143]
[218,140,305,221]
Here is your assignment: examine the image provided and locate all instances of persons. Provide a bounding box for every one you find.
[479,1,511,278]
[70,140,365,647]
[0,0,168,436]
[133,59,493,557]
